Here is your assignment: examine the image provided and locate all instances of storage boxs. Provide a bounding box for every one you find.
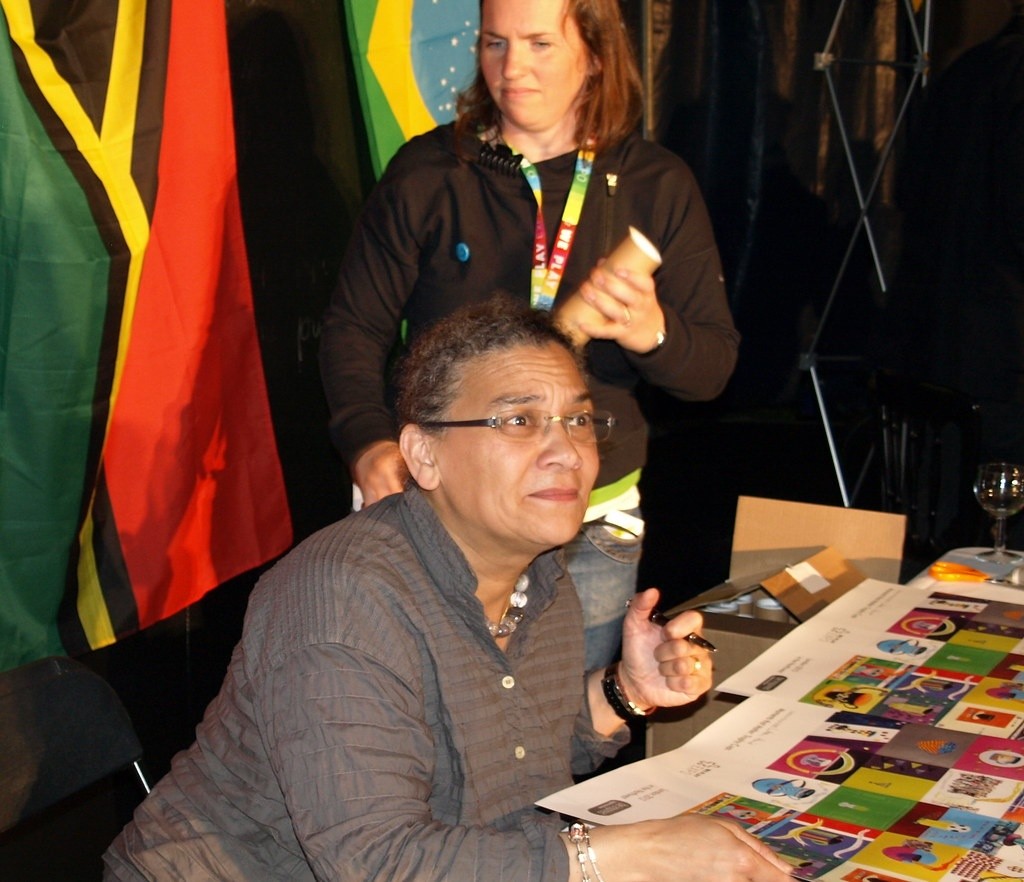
[645,495,908,761]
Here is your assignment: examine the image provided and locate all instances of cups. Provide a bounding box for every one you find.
[557,224,662,347]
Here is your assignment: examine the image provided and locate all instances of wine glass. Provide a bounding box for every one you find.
[973,463,1024,565]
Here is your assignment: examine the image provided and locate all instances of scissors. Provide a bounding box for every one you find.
[928,560,1004,586]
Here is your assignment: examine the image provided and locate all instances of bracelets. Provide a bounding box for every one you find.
[602,662,656,726]
[569,823,603,882]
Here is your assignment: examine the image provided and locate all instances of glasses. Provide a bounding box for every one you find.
[417,408,618,444]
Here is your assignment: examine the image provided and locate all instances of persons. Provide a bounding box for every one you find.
[85,291,720,881]
[302,1,743,777]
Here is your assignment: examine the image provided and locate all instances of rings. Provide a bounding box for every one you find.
[690,655,701,675]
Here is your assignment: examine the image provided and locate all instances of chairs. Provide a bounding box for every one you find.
[0,656,151,881]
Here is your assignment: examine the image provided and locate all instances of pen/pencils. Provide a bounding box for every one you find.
[623,597,719,652]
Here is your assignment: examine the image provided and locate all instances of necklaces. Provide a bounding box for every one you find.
[482,571,530,638]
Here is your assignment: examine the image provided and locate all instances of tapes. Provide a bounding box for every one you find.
[703,595,788,622]
[554,225,662,352]
